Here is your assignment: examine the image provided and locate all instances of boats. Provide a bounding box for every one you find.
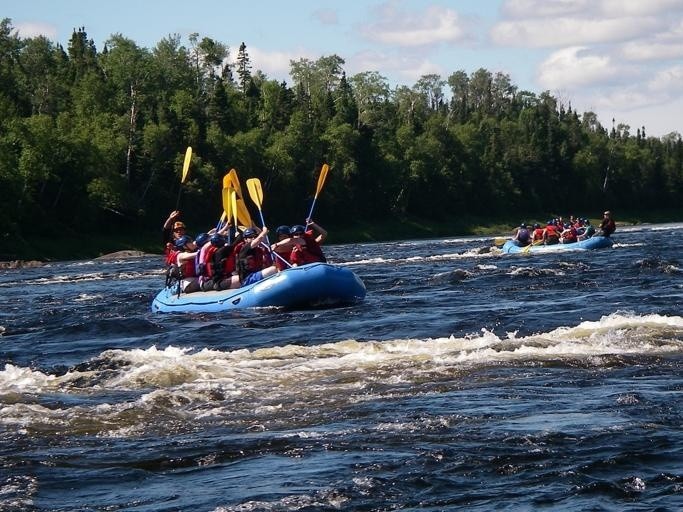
[500,235,614,256]
[148,258,373,320]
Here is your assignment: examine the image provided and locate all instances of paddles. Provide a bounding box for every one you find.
[169,147,192,247]
[216,169,253,245]
[494,239,509,245]
[522,243,532,252]
[306,164,329,232]
[246,178,275,264]
[532,239,543,246]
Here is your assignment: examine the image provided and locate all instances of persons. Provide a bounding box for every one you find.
[512,216,595,247]
[162,210,328,294]
[592,211,616,237]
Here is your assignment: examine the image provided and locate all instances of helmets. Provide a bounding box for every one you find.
[169,221,306,248]
[604,211,611,215]
[519,217,589,228]
